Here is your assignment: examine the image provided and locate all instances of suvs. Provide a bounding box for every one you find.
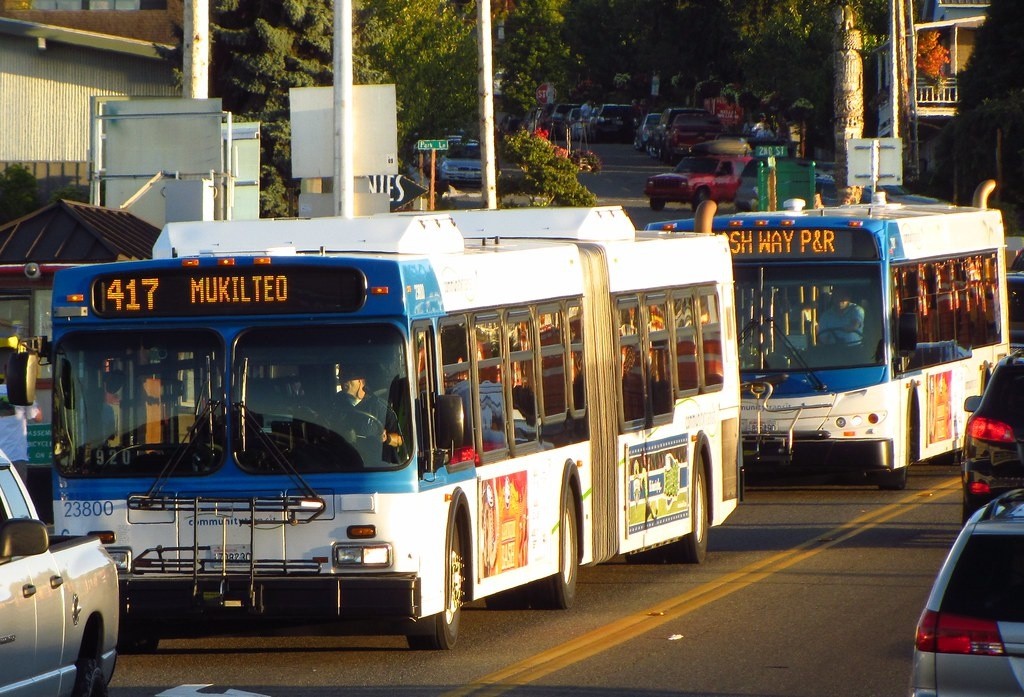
[642,152,758,211]
[958,343,1024,527]
[911,488,1024,696]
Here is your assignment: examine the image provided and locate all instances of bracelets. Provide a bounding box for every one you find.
[383,433,391,446]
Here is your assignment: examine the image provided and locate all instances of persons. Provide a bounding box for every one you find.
[751,115,771,132]
[581,100,594,137]
[0,361,41,485]
[317,364,404,465]
[756,123,775,138]
[817,285,864,346]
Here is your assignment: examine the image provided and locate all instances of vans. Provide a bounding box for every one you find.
[734,158,949,217]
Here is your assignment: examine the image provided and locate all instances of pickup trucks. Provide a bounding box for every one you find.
[0,449,124,697]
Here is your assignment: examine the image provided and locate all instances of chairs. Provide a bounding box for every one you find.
[452,338,724,424]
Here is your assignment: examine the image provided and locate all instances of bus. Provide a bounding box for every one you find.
[3,199,749,655]
[645,181,1016,492]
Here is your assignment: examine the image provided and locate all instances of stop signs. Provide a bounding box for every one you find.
[536,84,558,105]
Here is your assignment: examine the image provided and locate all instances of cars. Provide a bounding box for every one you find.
[408,101,804,185]
